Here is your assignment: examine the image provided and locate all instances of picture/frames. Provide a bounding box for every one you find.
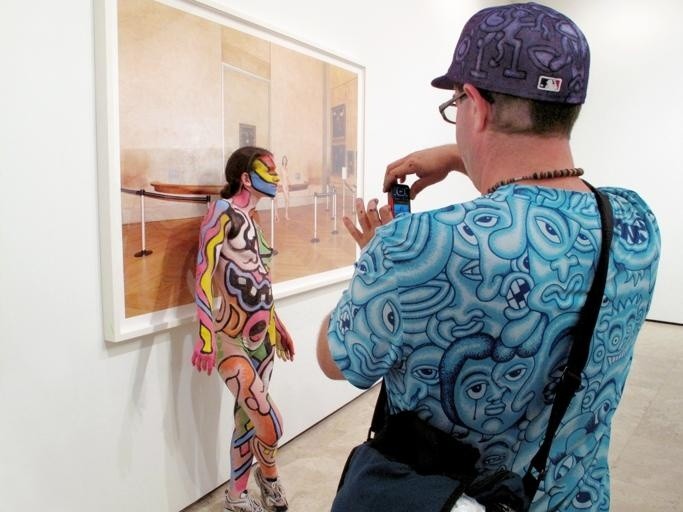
[93,1,364,345]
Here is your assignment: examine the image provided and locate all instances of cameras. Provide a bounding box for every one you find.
[389,184,410,218]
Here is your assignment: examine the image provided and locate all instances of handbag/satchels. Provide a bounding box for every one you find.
[331,446,531,511]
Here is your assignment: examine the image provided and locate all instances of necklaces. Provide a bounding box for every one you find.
[480,164,587,197]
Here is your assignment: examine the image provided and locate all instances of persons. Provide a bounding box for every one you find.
[185,145,295,512]
[312,0,664,512]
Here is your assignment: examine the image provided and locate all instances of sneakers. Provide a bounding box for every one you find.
[223,488,267,511]
[254,467,288,512]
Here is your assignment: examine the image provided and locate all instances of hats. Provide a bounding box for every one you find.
[431,1,590,106]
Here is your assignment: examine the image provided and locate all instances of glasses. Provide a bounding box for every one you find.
[439,91,495,124]
